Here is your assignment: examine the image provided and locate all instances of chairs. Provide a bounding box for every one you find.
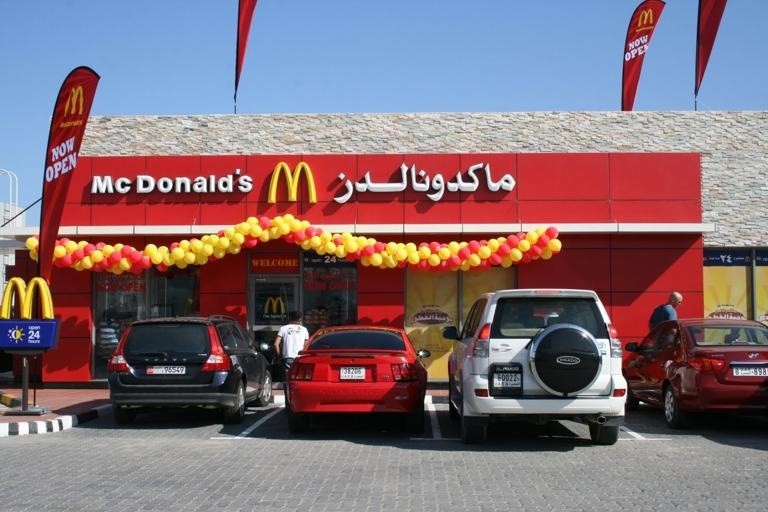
[504,316,569,328]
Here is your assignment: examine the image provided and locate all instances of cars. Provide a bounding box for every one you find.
[279,323,432,437]
[620,316,767,429]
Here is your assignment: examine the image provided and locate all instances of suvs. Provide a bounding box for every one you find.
[439,286,630,446]
[106,313,274,430]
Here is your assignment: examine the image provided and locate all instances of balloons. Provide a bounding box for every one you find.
[269,214,561,273]
[25,218,272,275]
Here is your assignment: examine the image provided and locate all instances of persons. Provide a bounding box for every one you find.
[274,309,311,412]
[649,291,683,340]
[98,307,120,358]
